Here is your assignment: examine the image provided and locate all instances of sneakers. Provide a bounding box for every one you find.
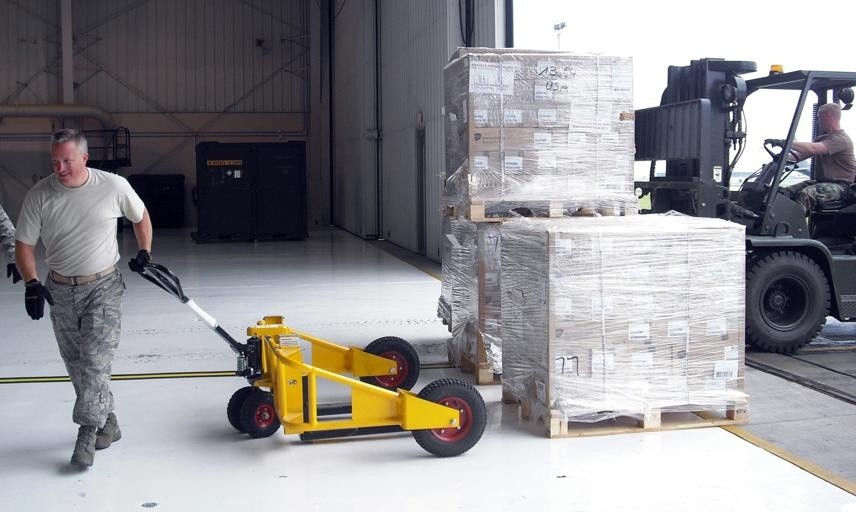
[94,413,121,451]
[70,424,97,468]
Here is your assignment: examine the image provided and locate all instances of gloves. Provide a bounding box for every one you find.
[23,277,55,321]
[6,263,23,286]
[128,250,152,273]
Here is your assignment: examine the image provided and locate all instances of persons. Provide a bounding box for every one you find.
[0,206,22,285]
[766,102,855,221]
[12,127,154,469]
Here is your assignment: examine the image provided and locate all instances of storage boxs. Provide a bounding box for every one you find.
[501,212,745,402]
[442,221,502,382]
[442,50,635,218]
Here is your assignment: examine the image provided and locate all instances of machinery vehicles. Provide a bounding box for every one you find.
[635,57,856,354]
